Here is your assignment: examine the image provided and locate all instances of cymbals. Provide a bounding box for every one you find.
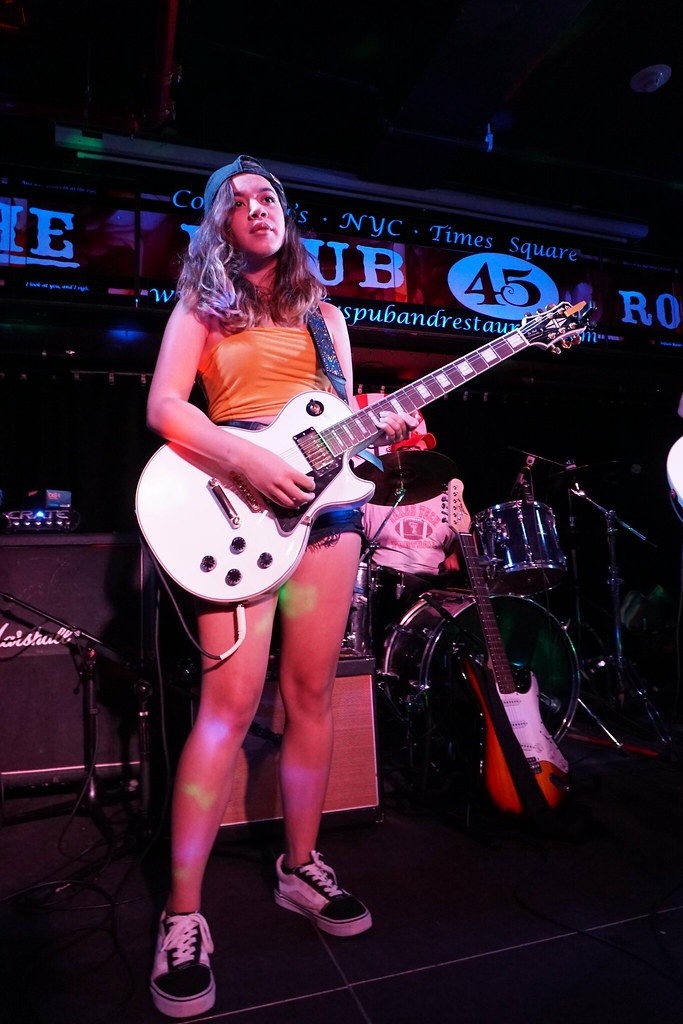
[353,449,458,508]
[535,459,626,490]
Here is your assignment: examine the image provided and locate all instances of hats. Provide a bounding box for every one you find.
[204,154,286,215]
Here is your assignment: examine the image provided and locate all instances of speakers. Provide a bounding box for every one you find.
[174,645,384,847]
[0,532,157,790]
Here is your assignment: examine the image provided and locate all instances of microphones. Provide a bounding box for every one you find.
[545,697,561,714]
[510,466,528,501]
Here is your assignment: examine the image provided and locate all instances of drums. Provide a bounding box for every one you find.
[471,500,568,597]
[376,589,581,777]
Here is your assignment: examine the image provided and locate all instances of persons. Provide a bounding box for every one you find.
[146,155,419,1018]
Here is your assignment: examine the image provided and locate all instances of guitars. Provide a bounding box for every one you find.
[440,477,572,816]
[135,300,596,607]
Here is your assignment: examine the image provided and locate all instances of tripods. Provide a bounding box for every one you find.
[562,475,683,771]
[0,587,288,907]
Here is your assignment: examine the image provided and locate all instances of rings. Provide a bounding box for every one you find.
[396,434,402,439]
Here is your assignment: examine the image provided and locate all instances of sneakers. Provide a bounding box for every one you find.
[150,908,215,1017]
[274,849,374,937]
